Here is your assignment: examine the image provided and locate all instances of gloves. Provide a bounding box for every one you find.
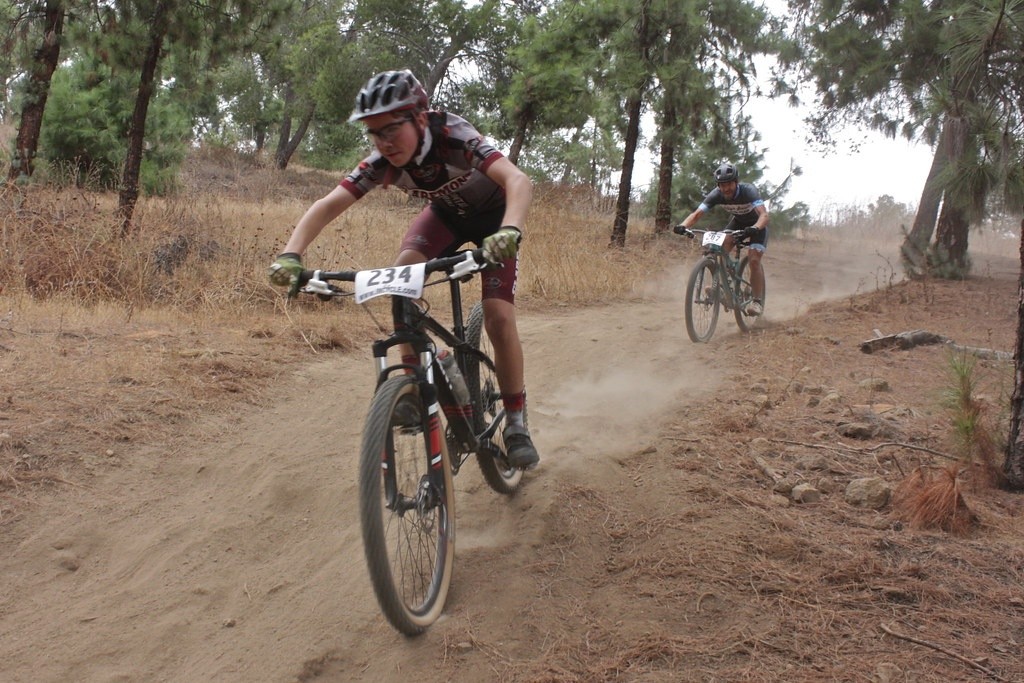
[482,229,521,271]
[742,226,756,244]
[269,256,305,298]
[674,224,686,234]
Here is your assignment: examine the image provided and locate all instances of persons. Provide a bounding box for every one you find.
[267,68,541,470]
[672,163,770,316]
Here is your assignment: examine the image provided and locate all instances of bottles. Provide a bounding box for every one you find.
[437,350,469,409]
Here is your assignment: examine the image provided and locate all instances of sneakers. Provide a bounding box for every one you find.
[747,300,764,315]
[706,286,725,302]
[503,426,540,467]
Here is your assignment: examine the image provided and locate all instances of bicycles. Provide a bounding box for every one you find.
[682,227,767,345]
[289,245,524,635]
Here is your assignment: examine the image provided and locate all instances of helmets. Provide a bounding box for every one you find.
[346,67,429,124]
[713,164,738,184]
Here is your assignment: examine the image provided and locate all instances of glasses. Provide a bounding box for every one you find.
[366,118,415,143]
